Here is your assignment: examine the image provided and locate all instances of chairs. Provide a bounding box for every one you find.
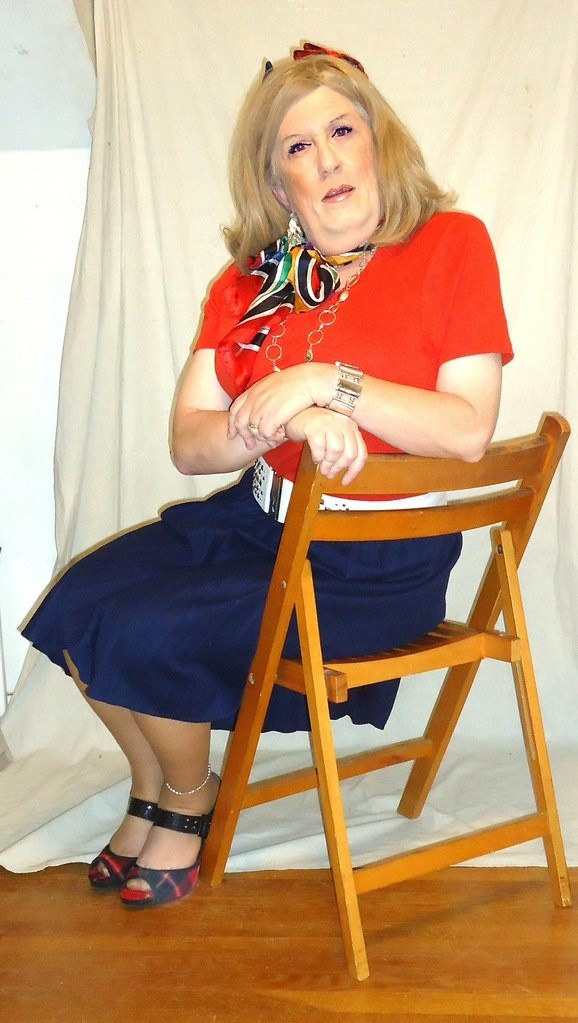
[196,412,572,982]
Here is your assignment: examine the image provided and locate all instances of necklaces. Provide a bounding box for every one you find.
[264,249,378,371]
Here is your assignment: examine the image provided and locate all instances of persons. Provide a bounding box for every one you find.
[22,44,512,906]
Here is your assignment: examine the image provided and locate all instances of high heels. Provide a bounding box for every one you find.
[119,770,223,909]
[88,794,161,885]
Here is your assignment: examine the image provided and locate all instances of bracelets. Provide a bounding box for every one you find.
[329,364,363,416]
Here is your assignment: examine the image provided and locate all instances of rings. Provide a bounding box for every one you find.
[249,424,258,428]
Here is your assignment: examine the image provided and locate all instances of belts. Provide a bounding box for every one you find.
[251,456,447,523]
